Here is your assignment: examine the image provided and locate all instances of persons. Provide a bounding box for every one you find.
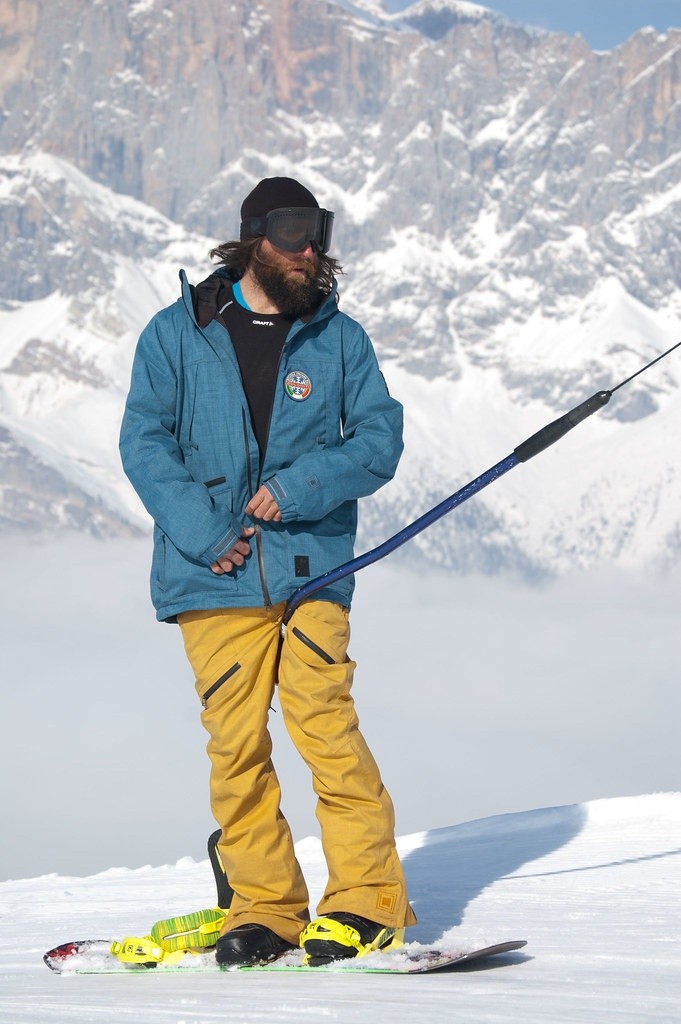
[119,176,419,968]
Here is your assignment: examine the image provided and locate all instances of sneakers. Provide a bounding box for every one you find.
[304,912,393,956]
[215,923,300,963]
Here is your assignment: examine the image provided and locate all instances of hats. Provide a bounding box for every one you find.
[241,177,319,221]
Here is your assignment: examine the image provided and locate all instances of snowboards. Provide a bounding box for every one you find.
[43,935,528,975]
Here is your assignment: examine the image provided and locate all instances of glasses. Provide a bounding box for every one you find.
[265,207,335,253]
